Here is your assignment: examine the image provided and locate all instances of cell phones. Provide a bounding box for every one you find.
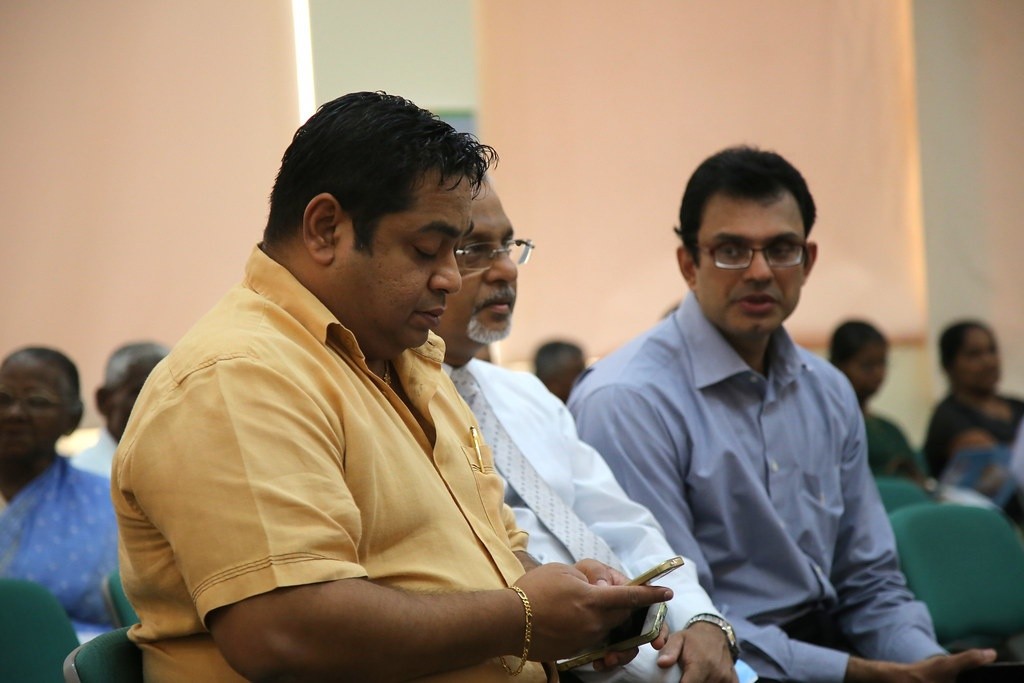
[556,602,669,672]
[624,557,685,587]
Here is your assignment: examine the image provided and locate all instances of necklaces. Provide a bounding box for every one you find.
[384,360,392,384]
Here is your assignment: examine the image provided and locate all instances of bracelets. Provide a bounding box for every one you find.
[683,614,739,664]
[499,586,532,676]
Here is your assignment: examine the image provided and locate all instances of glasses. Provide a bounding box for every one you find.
[456,239,535,270]
[695,243,805,269]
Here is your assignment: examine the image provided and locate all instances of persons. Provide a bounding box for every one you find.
[0,343,168,623]
[829,320,1024,528]
[564,147,997,683]
[535,342,584,404]
[431,169,758,683]
[110,90,673,683]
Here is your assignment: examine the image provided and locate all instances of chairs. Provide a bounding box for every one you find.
[101,568,142,628]
[874,476,937,523]
[0,577,82,683]
[62,626,144,683]
[888,503,1024,672]
